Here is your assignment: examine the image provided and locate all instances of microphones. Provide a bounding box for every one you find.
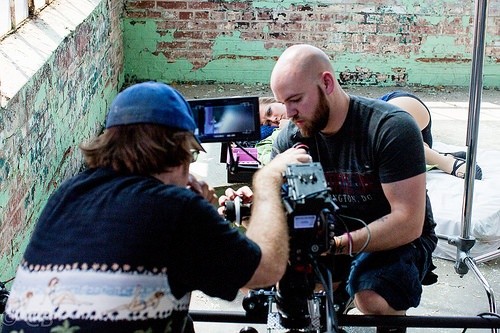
[292,142,309,155]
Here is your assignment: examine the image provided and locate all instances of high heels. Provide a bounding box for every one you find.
[444,150,482,179]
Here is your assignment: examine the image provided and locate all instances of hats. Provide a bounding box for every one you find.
[106,82,207,154]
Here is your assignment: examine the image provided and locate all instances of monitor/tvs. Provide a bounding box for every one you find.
[186,96,261,143]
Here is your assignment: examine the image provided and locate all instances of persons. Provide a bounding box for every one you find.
[0,82,312,333]
[218,44,438,333]
[260,91,482,178]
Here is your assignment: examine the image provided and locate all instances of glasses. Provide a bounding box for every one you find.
[188,148,199,161]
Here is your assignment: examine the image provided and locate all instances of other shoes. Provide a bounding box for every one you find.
[306,279,355,333]
[376,326,408,332]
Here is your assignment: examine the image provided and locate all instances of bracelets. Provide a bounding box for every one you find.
[345,232,353,254]
